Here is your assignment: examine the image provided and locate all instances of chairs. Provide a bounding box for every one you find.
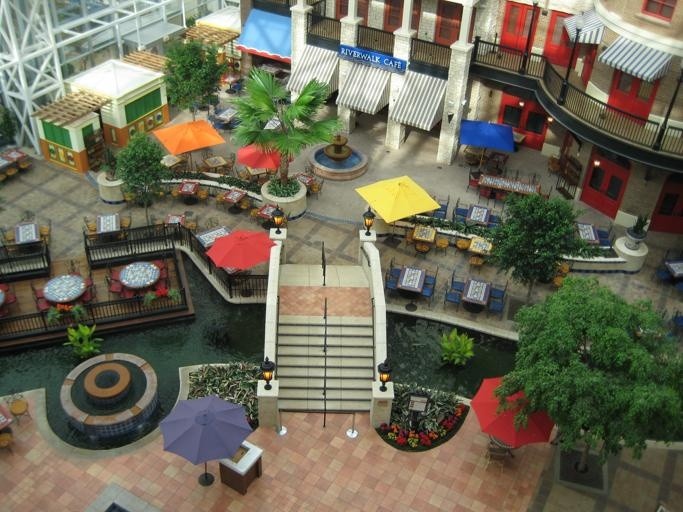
[382,194,616,320]
[121,149,324,228]
[484,443,506,475]
[0,146,32,184]
[650,248,683,334]
[0,213,249,317]
[4,389,32,425]
[460,146,561,206]
[207,80,279,131]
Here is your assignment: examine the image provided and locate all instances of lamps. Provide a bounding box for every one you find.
[259,356,275,390]
[376,356,393,391]
[362,206,375,235]
[270,204,285,236]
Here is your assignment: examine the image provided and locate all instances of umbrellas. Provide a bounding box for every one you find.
[159,398,253,476]
[236,143,294,170]
[459,119,514,168]
[471,378,555,448]
[205,230,277,272]
[153,120,225,169]
[354,175,441,241]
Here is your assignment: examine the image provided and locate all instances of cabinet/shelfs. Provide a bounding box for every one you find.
[554,154,581,199]
[83,128,109,172]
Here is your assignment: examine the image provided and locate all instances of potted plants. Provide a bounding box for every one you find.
[223,63,345,219]
[623,211,650,251]
[95,148,125,205]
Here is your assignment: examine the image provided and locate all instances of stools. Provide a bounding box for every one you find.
[0,433,15,454]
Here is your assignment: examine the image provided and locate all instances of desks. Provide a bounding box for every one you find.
[488,434,519,459]
[512,131,526,151]
[0,405,14,432]
[219,441,264,496]
